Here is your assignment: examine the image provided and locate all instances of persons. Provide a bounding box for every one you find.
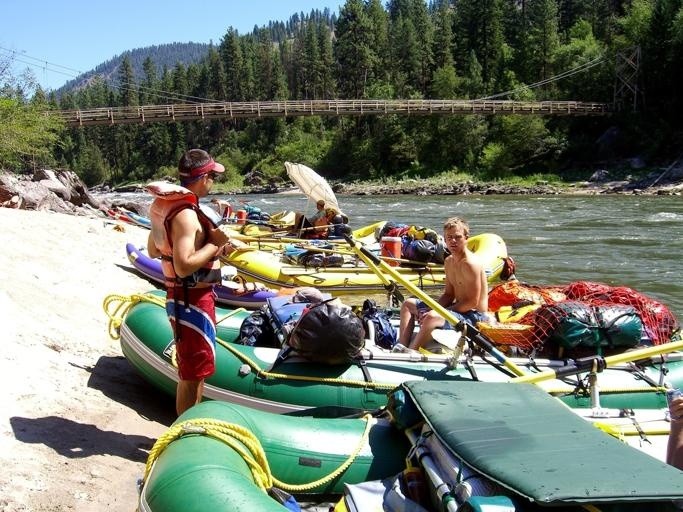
[295,200,326,234]
[209,198,232,218]
[667,389,683,471]
[148,150,232,417]
[393,217,489,351]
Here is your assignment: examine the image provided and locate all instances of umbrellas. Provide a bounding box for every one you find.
[285,161,340,236]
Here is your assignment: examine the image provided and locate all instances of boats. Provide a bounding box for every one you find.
[126,198,235,230]
[137,381,683,512]
[220,220,389,244]
[126,243,280,310]
[224,209,296,233]
[107,209,132,222]
[120,285,683,419]
[220,233,508,298]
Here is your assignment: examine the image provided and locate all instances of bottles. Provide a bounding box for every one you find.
[667,388,683,418]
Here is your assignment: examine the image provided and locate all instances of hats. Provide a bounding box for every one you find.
[316,200,325,207]
[178,154,225,178]
[326,208,336,215]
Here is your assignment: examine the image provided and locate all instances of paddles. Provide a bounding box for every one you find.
[606,426,670,438]
[251,224,337,238]
[279,265,444,277]
